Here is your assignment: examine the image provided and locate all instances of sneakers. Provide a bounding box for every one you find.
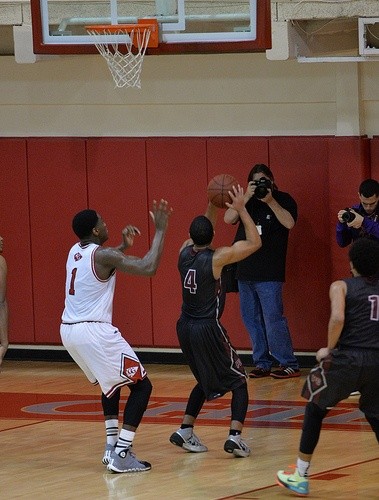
[276,465,308,496]
[224,435,252,458]
[270,365,300,378]
[169,428,208,452]
[107,450,151,473]
[247,367,270,378]
[102,445,112,464]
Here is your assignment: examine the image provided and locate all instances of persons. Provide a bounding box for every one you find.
[275,239,379,497]
[59,198,173,475]
[0,237,9,364]
[232,163,301,378]
[334,177,379,248]
[168,185,262,458]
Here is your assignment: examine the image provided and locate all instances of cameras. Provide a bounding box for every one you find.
[341,208,359,223]
[251,176,271,199]
